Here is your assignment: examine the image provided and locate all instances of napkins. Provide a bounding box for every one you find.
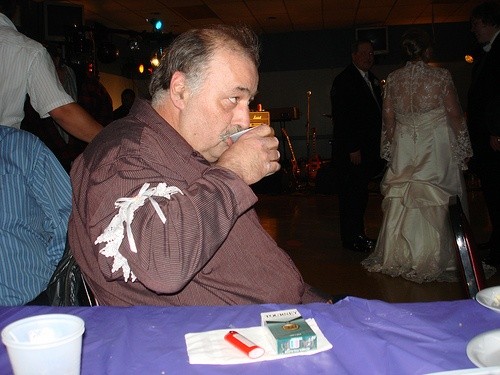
[185,318,333,364]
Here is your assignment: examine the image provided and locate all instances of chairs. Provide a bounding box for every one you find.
[449,195,486,299]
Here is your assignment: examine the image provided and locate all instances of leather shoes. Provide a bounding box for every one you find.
[352,232,377,249]
[343,240,369,253]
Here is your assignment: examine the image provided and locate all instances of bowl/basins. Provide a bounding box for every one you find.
[475,287,500,311]
[467,328,500,375]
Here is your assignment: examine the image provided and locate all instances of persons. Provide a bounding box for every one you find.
[330,39,387,253]
[113,89,136,120]
[23,46,113,172]
[466,2,500,251]
[0,0,104,143]
[68,27,327,307]
[359,29,473,284]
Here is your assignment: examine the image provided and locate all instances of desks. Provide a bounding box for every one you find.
[0,296,500,375]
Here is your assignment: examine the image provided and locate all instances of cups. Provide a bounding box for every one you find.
[229,126,261,143]
[0,312,85,375]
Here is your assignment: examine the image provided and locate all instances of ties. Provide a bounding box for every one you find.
[364,73,382,112]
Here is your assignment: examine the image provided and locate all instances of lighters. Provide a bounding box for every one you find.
[224,330,265,360]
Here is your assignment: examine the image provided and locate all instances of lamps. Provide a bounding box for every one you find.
[150,17,162,31]
